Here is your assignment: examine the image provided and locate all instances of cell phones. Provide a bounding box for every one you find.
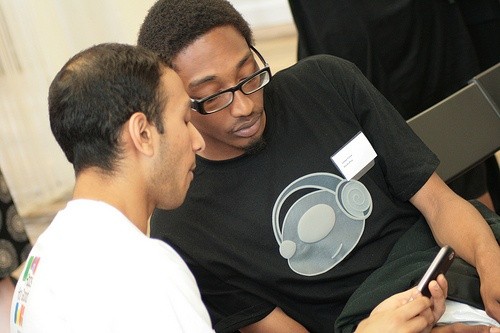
[407,245,456,302]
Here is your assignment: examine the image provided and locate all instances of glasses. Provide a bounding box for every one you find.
[178,45,274,114]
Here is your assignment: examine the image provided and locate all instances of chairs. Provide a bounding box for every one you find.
[405,79,500,183]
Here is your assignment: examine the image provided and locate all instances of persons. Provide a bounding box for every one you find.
[131,0,500,333]
[7,40,450,333]
[287,0,498,214]
